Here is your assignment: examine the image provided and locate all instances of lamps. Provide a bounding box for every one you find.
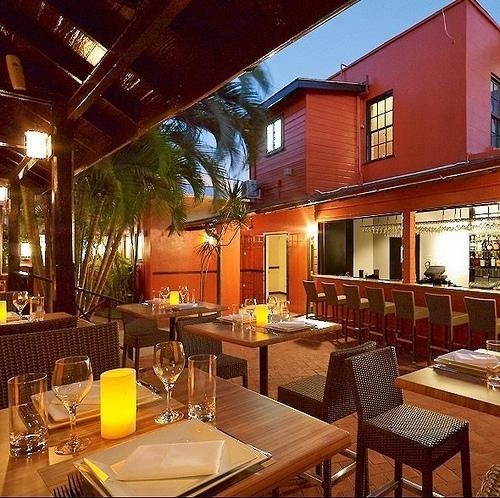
[21,130,55,161]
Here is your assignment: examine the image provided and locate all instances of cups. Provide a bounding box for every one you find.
[152,341,185,428]
[485,338,500,392]
[51,356,93,455]
[28,296,46,320]
[278,300,291,324]
[176,283,189,306]
[159,284,171,311]
[266,296,277,324]
[186,353,218,425]
[243,297,257,328]
[13,288,29,320]
[8,372,48,456]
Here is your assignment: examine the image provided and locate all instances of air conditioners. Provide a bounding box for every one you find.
[239,179,263,201]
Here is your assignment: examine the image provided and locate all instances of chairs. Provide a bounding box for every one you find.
[177,312,249,391]
[0,321,122,411]
[391,289,430,363]
[340,280,373,341]
[0,318,80,333]
[277,341,378,498]
[320,282,348,340]
[463,296,500,351]
[302,281,326,322]
[364,284,399,346]
[345,346,472,498]
[422,292,470,366]
[121,312,172,377]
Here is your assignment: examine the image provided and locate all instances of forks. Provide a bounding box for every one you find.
[67,470,86,498]
[53,484,71,498]
